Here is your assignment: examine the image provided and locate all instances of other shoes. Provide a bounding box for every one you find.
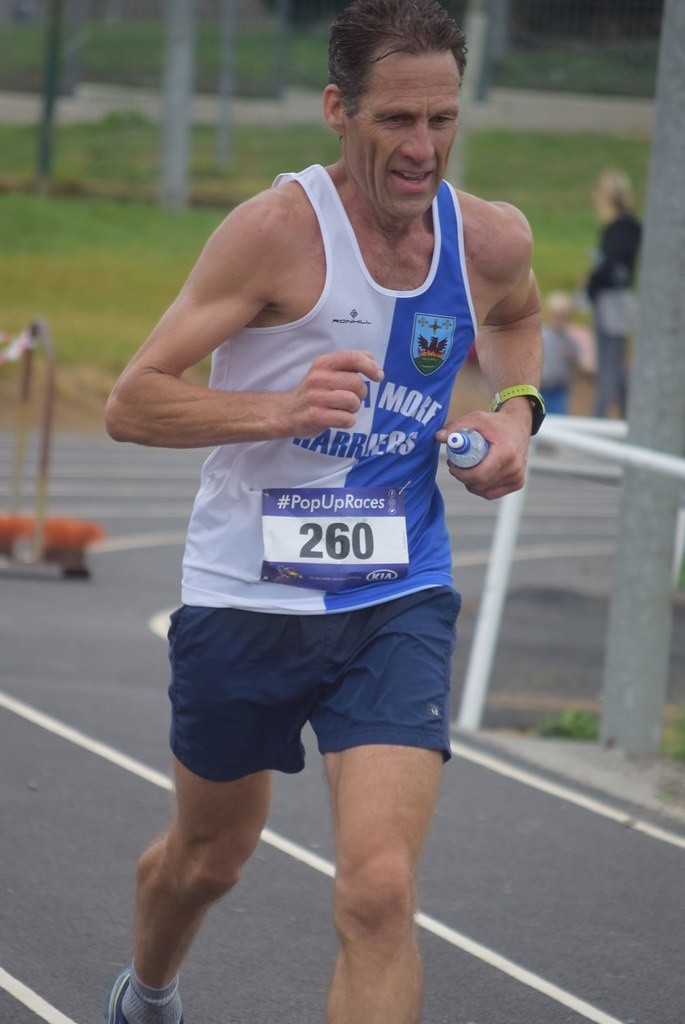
[104,967,185,1024]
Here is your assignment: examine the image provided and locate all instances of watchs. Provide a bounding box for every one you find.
[489,384,546,436]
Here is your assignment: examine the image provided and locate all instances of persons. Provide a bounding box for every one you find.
[542,180,641,419]
[105,1,546,1024]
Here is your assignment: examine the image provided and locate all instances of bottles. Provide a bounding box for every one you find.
[446,428,489,468]
